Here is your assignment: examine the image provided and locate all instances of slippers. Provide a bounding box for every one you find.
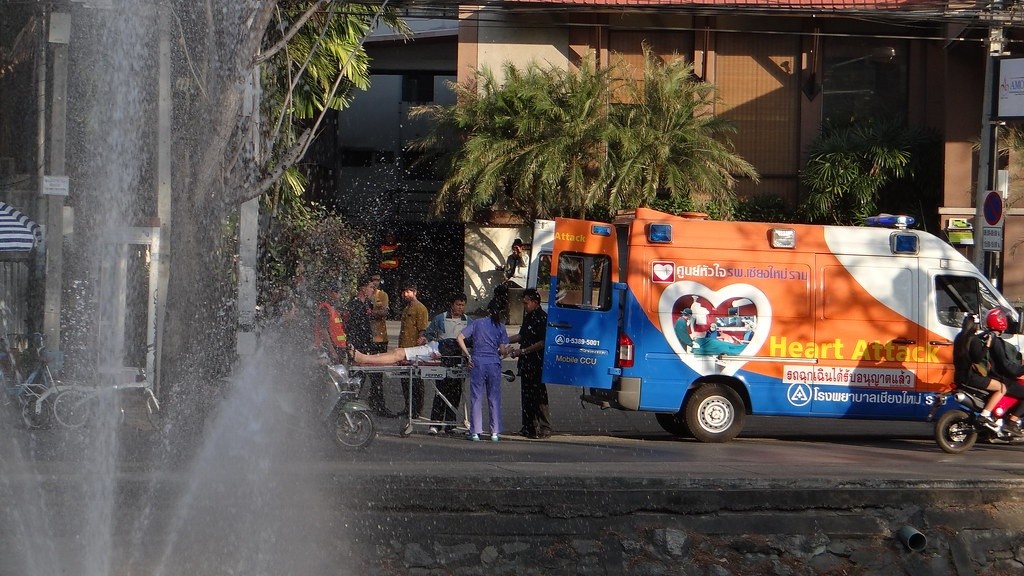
[347,344,359,364]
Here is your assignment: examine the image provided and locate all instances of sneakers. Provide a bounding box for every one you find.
[491,433,502,442]
[977,414,998,428]
[1002,421,1024,437]
[466,434,481,442]
[427,427,439,435]
[446,428,467,436]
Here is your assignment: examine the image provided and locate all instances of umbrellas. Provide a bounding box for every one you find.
[0,202,45,261]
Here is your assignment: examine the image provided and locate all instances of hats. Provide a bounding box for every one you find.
[513,239,523,248]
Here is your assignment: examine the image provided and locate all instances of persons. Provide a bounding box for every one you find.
[480,241,530,313]
[397,280,425,417]
[674,309,700,352]
[457,300,510,441]
[346,338,473,366]
[508,287,552,441]
[267,269,396,418]
[706,323,717,340]
[953,308,1024,436]
[417,293,473,437]
[740,321,755,344]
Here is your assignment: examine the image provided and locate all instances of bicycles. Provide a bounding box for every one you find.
[1,327,164,432]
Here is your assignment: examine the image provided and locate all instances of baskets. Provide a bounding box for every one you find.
[44,351,64,371]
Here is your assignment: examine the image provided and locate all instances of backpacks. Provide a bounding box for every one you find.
[997,337,1023,379]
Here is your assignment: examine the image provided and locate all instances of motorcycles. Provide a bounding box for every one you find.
[930,379,1023,454]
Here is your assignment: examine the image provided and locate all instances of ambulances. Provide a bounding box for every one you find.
[524,211,1024,444]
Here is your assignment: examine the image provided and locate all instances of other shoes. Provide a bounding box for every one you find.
[376,405,397,418]
[519,426,537,437]
[534,429,554,439]
[397,408,421,419]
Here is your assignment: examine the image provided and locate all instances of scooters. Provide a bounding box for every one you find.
[307,346,378,451]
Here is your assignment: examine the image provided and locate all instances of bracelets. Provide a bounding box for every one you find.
[467,355,471,358]
[506,349,508,354]
[521,348,525,355]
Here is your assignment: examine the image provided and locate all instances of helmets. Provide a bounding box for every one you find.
[986,306,1007,331]
